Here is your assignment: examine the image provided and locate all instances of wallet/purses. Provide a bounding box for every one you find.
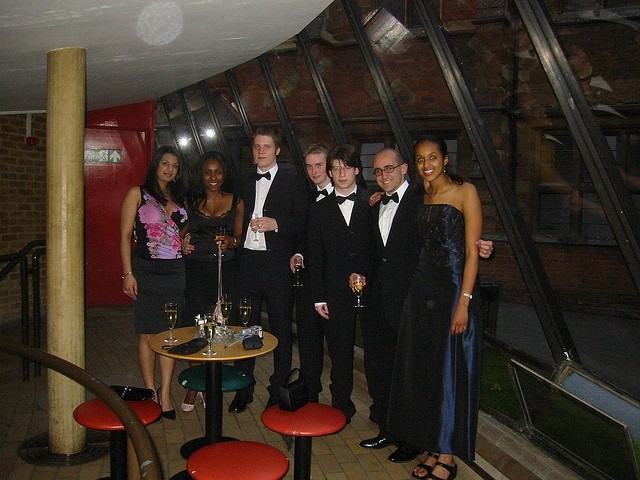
[168,338,208,354]
[243,335,263,350]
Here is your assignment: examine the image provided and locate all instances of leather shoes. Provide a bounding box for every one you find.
[359,435,399,449]
[228,391,253,413]
[387,445,425,464]
[265,396,278,409]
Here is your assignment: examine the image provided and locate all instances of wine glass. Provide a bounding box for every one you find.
[351,274,366,308]
[163,303,179,343]
[202,312,218,356]
[213,228,225,257]
[218,295,232,338]
[292,255,304,288]
[249,212,260,243]
[238,299,252,335]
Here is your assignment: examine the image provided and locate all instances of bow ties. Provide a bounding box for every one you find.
[336,192,356,205]
[312,189,328,198]
[256,170,271,181]
[381,192,398,205]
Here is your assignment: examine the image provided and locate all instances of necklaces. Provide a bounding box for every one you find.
[204,196,222,206]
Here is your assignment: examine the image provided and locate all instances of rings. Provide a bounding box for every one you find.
[258,225,261,227]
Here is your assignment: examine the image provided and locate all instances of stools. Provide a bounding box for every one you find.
[178,364,254,461]
[73,397,162,480]
[261,402,347,480]
[169,441,290,480]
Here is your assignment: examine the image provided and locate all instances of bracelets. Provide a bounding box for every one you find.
[459,290,478,301]
[120,270,135,281]
[232,236,237,248]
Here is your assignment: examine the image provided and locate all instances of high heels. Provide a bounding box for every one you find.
[156,387,175,420]
[199,392,206,409]
[181,392,199,412]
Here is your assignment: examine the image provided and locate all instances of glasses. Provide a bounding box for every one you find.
[372,163,403,176]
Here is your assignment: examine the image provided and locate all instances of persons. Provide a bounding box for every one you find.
[183,152,243,412]
[291,145,338,408]
[411,135,483,480]
[306,141,386,433]
[358,148,492,464]
[230,126,307,412]
[120,147,190,420]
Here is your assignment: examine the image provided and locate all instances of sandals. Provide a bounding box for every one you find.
[425,462,457,480]
[411,451,439,480]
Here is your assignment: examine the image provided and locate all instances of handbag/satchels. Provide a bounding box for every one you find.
[109,384,155,402]
[278,368,309,411]
